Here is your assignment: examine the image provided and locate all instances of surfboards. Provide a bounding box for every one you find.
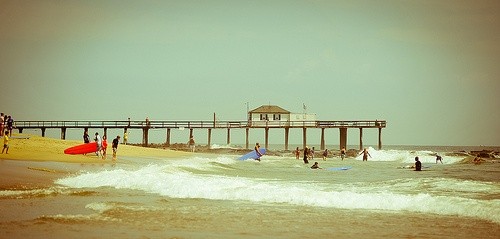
[327,166,350,171]
[239,148,265,160]
[64,142,108,155]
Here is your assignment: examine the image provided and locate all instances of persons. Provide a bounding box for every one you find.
[303,146,310,164]
[187,135,196,152]
[83,131,90,155]
[111,136,121,158]
[435,154,443,164]
[322,149,328,160]
[0,112,14,137]
[311,162,319,169]
[101,135,108,154]
[415,157,422,171]
[124,130,128,145]
[341,146,346,160]
[295,147,300,159]
[308,147,315,160]
[94,132,101,156]
[358,148,372,162]
[255,142,262,161]
[2,131,10,154]
